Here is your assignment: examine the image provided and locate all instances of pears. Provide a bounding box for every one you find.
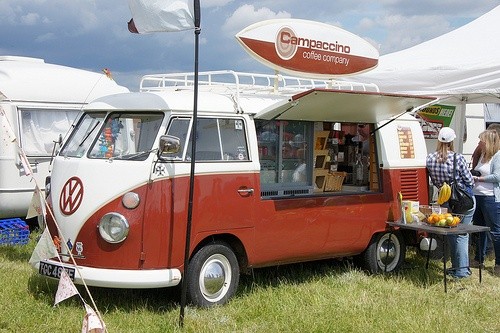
[435,222,440,226]
[440,219,447,226]
[446,216,453,223]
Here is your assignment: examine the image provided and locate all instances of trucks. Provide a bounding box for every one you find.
[32,70,440,310]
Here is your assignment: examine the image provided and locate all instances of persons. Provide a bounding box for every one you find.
[425,127,475,278]
[468,130,500,271]
[471,123,500,170]
[282,142,292,158]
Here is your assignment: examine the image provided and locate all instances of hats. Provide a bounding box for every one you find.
[438,127,456,142]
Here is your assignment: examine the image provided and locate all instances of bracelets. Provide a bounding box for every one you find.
[476,177,479,183]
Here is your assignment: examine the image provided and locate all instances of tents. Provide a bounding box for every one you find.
[332,4,500,157]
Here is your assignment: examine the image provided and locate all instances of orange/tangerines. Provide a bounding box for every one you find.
[427,213,460,227]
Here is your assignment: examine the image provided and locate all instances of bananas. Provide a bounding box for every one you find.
[437,182,451,204]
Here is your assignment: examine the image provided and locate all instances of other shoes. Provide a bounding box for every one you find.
[469,259,484,268]
[494,265,500,272]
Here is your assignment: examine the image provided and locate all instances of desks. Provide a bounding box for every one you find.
[384,222,491,294]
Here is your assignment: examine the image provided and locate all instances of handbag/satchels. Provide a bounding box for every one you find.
[448,178,474,214]
